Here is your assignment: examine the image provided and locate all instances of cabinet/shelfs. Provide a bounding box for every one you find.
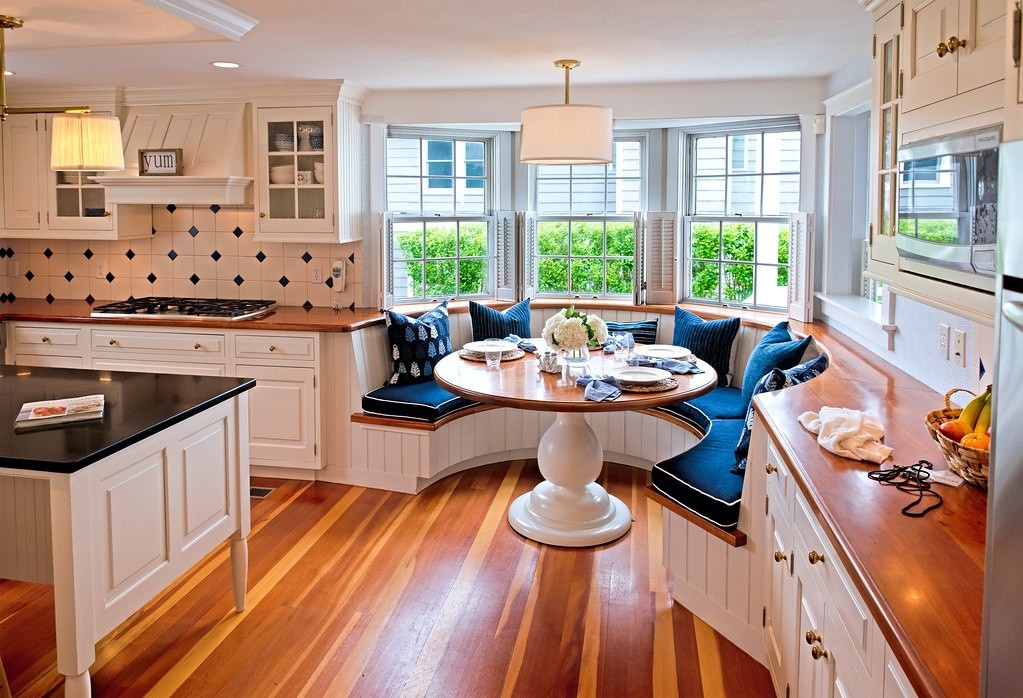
[226,331,318,466]
[3,103,114,232]
[12,320,82,367]
[870,0,1023,264]
[761,429,919,698]
[257,105,334,234]
[87,324,227,377]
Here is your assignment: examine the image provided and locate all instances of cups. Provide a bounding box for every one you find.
[484,338,501,367]
[622,351,639,370]
[613,332,629,358]
[312,208,325,219]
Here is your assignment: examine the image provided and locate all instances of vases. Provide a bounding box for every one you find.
[563,343,591,368]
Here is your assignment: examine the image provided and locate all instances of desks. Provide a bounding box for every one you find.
[433,343,719,549]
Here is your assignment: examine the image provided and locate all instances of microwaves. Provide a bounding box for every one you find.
[896,125,1000,277]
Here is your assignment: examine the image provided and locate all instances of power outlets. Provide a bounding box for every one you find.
[936,324,950,359]
[96,260,107,279]
[312,266,323,283]
[8,259,18,277]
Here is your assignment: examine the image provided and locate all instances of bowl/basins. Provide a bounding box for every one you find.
[270,165,295,184]
[313,162,324,183]
[272,125,323,151]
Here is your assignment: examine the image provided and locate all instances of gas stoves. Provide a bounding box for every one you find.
[91,297,277,321]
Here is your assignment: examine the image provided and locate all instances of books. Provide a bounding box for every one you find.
[14,394,104,428]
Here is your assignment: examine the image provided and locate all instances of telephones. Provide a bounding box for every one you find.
[331,259,345,292]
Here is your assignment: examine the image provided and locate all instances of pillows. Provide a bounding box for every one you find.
[730,355,826,473]
[376,300,451,384]
[674,305,741,387]
[603,316,657,343]
[741,321,813,407]
[468,296,532,342]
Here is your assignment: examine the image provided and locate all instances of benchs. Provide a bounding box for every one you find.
[349,323,747,655]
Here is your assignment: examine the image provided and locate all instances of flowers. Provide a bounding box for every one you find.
[539,303,609,349]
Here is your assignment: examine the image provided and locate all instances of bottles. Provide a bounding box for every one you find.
[544,351,557,370]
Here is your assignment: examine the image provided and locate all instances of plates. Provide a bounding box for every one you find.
[633,344,691,359]
[608,367,672,384]
[463,341,518,355]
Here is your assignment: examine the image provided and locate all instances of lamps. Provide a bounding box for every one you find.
[520,60,614,166]
[0,14,126,171]
